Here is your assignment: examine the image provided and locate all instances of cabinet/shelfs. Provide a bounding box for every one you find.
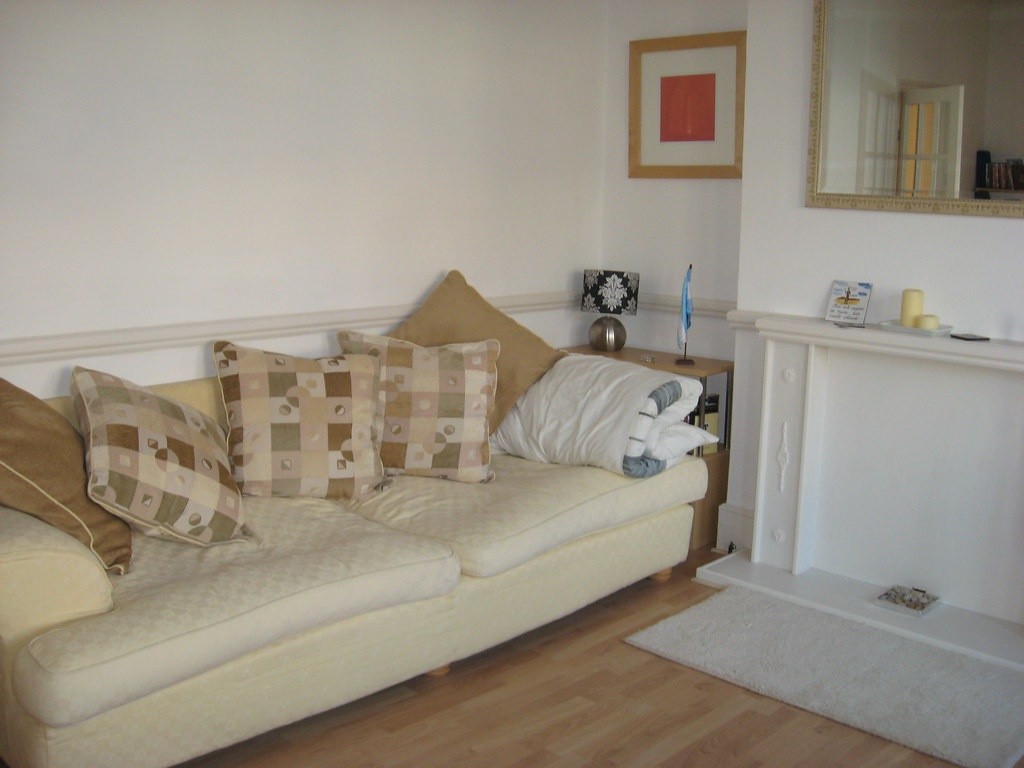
[691,310,1024,670]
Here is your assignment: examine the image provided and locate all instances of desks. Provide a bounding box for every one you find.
[560,344,734,552]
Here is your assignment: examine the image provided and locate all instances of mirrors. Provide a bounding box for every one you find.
[804,0,1024,218]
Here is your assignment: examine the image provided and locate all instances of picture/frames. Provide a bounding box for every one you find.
[628,29,748,180]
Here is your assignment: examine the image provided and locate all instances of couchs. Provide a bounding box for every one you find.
[0,376,709,768]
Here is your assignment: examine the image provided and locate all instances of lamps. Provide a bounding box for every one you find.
[580,269,640,352]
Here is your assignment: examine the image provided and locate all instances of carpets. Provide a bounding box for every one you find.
[622,583,1024,768]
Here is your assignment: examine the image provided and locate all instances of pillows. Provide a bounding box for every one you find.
[0,270,569,576]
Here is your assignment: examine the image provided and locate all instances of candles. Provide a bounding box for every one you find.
[900,288,939,331]
